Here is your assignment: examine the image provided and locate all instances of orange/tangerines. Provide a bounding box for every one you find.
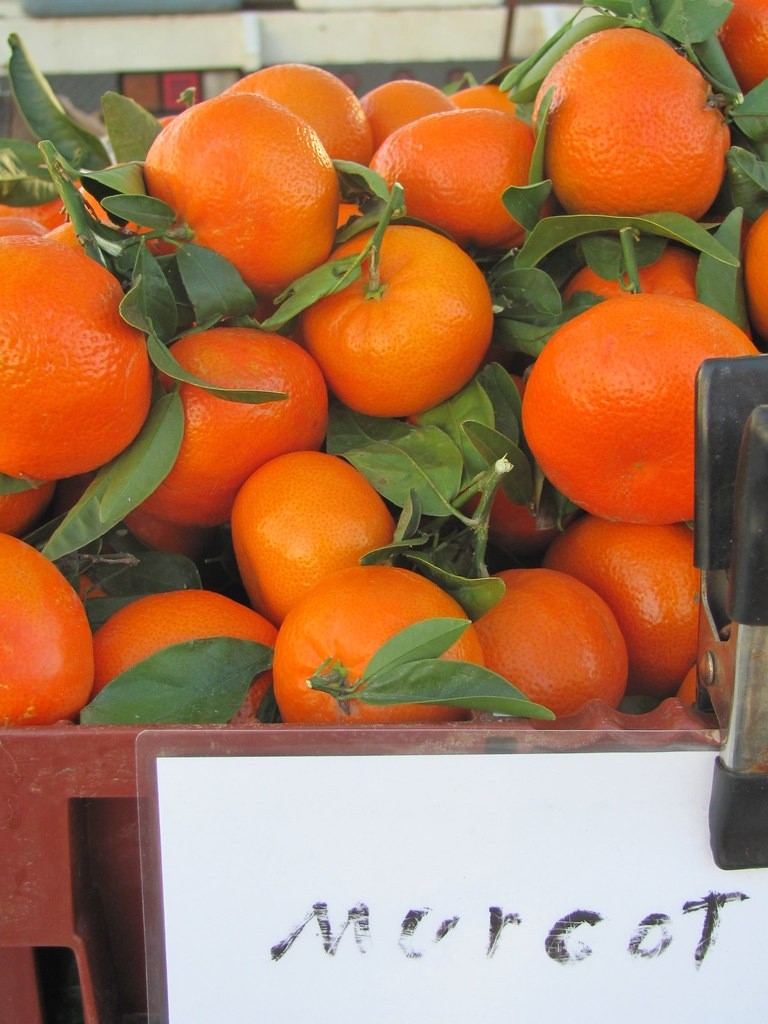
[0,0,768,732]
